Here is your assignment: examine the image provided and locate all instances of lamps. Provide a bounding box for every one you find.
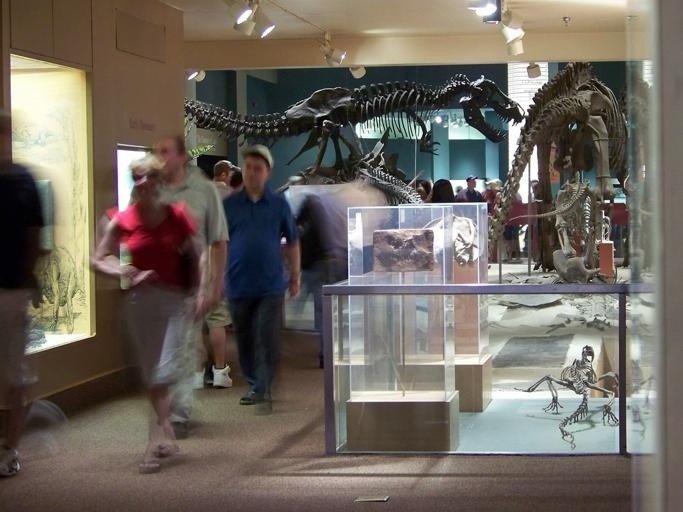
[183,64,208,85]
[466,1,526,60]
[526,60,540,78]
[347,65,367,80]
[224,0,346,67]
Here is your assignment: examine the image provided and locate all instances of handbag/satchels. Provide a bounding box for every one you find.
[165,202,200,294]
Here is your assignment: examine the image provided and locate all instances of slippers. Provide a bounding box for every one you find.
[155,440,181,458]
[138,457,161,475]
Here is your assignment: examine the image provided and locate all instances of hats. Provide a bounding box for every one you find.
[466,175,478,183]
[241,144,273,168]
[214,160,242,178]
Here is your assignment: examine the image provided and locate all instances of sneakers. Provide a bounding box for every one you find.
[211,362,235,389]
[239,385,272,415]
[193,367,205,391]
[170,422,189,439]
[0,446,22,475]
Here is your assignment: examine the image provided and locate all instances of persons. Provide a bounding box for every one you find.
[0,110,53,479]
[413,176,542,266]
